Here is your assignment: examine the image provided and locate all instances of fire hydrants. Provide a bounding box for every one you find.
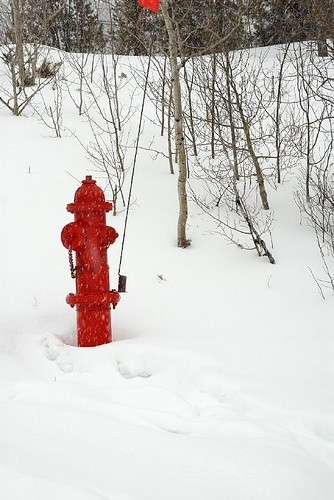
[60,175,121,348]
